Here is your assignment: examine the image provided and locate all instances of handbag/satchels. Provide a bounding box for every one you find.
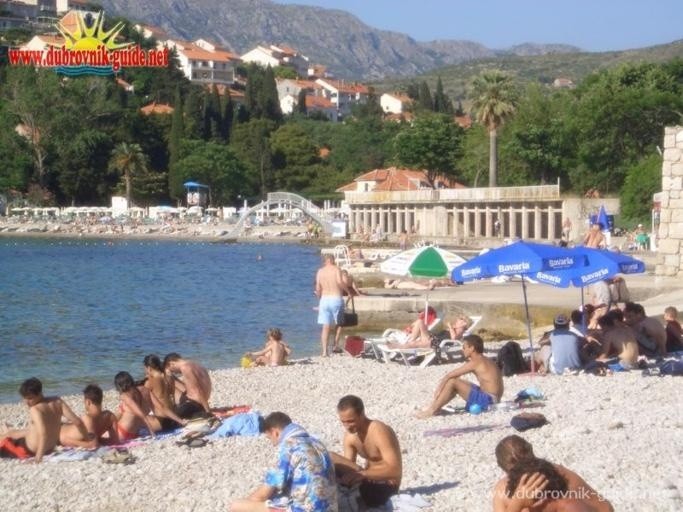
[338,312,357,327]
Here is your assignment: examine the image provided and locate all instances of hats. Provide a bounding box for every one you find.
[555,314,569,325]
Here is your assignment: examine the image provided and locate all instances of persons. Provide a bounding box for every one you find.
[386,314,473,351]
[310,247,364,355]
[493,220,502,235]
[388,278,461,294]
[230,411,340,512]
[257,329,292,364]
[245,329,284,368]
[585,224,606,250]
[327,395,402,509]
[561,218,573,240]
[493,432,614,512]
[534,279,683,378]
[615,223,648,250]
[416,335,504,421]
[505,459,598,512]
[0,353,213,463]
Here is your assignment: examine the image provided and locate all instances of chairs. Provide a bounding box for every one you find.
[378,317,481,367]
[346,317,441,360]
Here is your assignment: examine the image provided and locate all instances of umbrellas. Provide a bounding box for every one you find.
[524,235,644,332]
[381,244,470,329]
[590,205,609,236]
[451,237,589,377]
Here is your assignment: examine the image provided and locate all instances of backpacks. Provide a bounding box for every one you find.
[498,342,526,376]
